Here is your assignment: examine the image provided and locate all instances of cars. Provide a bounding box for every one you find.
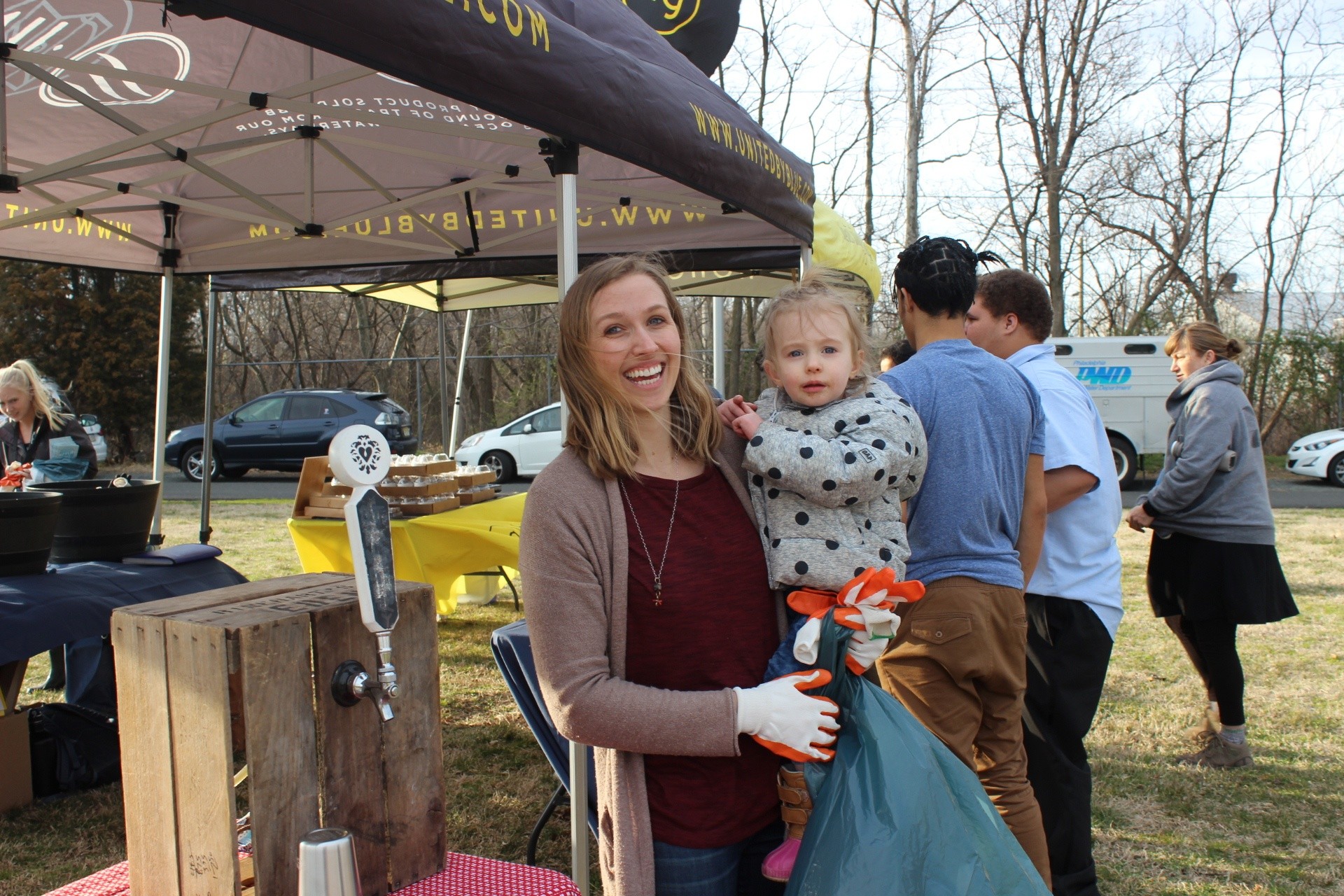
[1286,427,1344,488]
[453,401,570,485]
[0,411,108,461]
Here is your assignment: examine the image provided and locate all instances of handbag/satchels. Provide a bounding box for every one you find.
[22,703,121,797]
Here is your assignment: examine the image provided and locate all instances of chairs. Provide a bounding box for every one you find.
[487,620,605,864]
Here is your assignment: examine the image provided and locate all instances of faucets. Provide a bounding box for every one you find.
[332,660,399,724]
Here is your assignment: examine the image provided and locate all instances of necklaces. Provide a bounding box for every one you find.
[619,438,679,607]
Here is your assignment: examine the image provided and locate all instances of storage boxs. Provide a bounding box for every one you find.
[305,460,497,517]
[0,709,32,812]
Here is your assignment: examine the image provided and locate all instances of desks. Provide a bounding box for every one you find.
[0,556,257,709]
[287,491,533,617]
[40,816,586,896]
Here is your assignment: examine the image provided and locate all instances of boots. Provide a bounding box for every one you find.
[761,766,814,881]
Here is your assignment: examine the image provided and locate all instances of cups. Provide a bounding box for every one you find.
[297,828,363,896]
[1171,435,1237,471]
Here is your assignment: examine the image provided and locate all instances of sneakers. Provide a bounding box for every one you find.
[1183,707,1224,743]
[1176,731,1254,769]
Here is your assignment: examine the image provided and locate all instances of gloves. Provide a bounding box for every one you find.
[734,668,840,762]
[786,566,926,677]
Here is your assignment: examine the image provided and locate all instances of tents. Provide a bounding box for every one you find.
[0,0,878,896]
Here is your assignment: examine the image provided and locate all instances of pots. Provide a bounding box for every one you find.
[24,477,161,563]
[0,492,63,576]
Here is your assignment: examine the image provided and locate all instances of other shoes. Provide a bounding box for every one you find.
[26,676,65,693]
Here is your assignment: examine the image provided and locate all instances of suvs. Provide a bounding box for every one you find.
[164,387,418,483]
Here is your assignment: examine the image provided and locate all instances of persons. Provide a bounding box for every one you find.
[0,360,96,487]
[489,230,1303,896]
[117,425,135,458]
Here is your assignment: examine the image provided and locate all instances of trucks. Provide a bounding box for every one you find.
[1047,335,1181,490]
